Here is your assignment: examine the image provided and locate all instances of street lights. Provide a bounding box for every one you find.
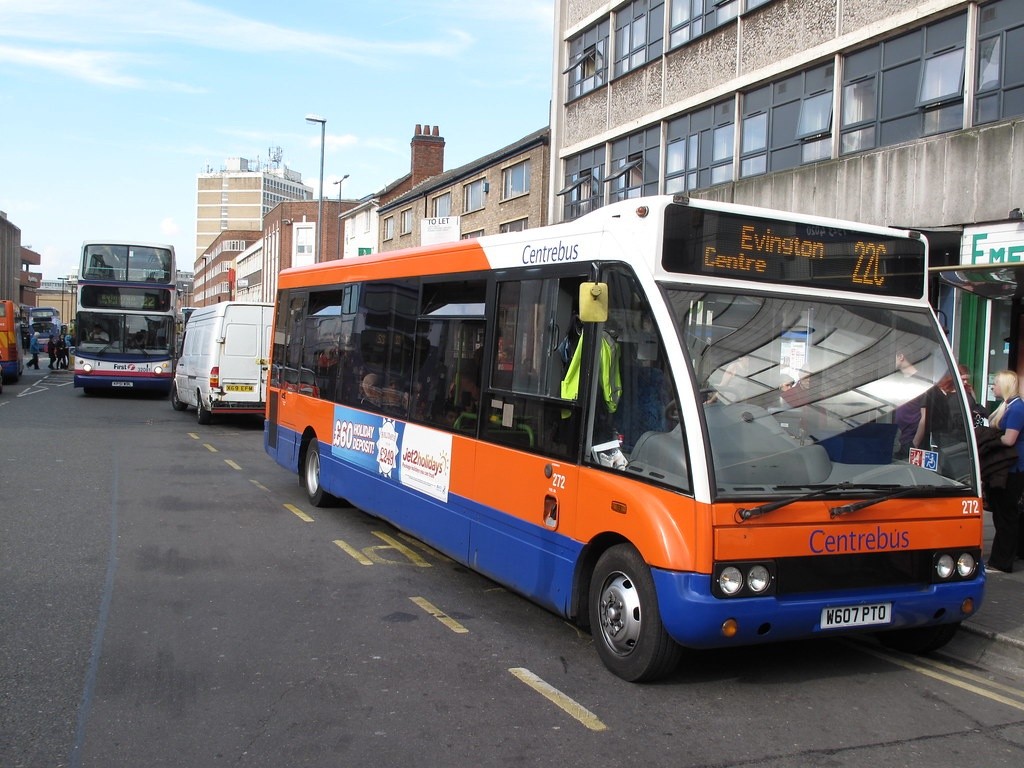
[305,113,327,262]
[333,174,350,260]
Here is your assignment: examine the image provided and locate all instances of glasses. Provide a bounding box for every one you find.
[389,381,401,386]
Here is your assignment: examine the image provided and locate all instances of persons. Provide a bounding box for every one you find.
[26,330,75,370]
[134,332,147,348]
[315,346,489,429]
[703,355,750,405]
[779,364,828,438]
[929,364,986,440]
[92,325,110,344]
[894,342,928,460]
[976,371,1024,572]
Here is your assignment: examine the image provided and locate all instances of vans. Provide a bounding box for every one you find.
[171,300,275,426]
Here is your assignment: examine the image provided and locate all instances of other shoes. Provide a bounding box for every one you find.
[984,564,1003,573]
[57,368,59,370]
[26,363,29,368]
[65,365,68,369]
[48,365,55,370]
[35,367,39,369]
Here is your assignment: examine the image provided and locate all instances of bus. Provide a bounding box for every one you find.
[73,239,178,400]
[29,306,61,353]
[18,305,34,349]
[180,306,201,325]
[261,190,989,688]
[0,299,24,383]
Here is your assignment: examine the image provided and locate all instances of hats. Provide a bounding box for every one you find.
[34,332,40,336]
